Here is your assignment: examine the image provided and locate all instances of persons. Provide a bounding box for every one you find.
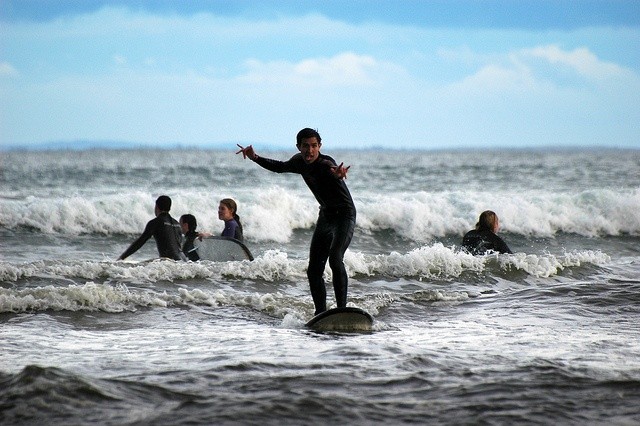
[463,210,512,256]
[235,128,356,316]
[116,195,182,261]
[178,214,199,261]
[199,199,243,243]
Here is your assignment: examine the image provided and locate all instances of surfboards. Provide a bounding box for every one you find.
[194,235,256,263]
[304,305,375,332]
